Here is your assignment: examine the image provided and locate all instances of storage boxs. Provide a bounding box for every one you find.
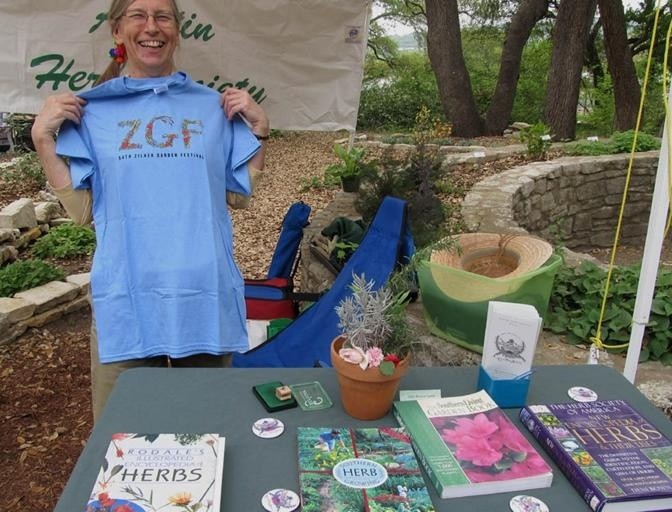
[417,231,565,353]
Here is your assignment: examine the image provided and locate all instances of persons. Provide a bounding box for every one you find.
[30,0,271,429]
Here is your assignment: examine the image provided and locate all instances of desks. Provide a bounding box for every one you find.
[56,367,671,512]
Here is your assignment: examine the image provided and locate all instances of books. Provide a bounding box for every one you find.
[391,388,554,500]
[85,432,228,512]
[294,424,435,512]
[519,399,672,511]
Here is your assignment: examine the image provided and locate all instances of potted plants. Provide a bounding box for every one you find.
[330,270,412,418]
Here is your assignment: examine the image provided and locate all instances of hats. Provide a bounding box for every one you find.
[429,232,553,304]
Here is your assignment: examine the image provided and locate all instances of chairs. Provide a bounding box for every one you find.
[234,195,407,370]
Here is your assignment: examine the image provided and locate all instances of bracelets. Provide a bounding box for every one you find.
[253,134,269,141]
